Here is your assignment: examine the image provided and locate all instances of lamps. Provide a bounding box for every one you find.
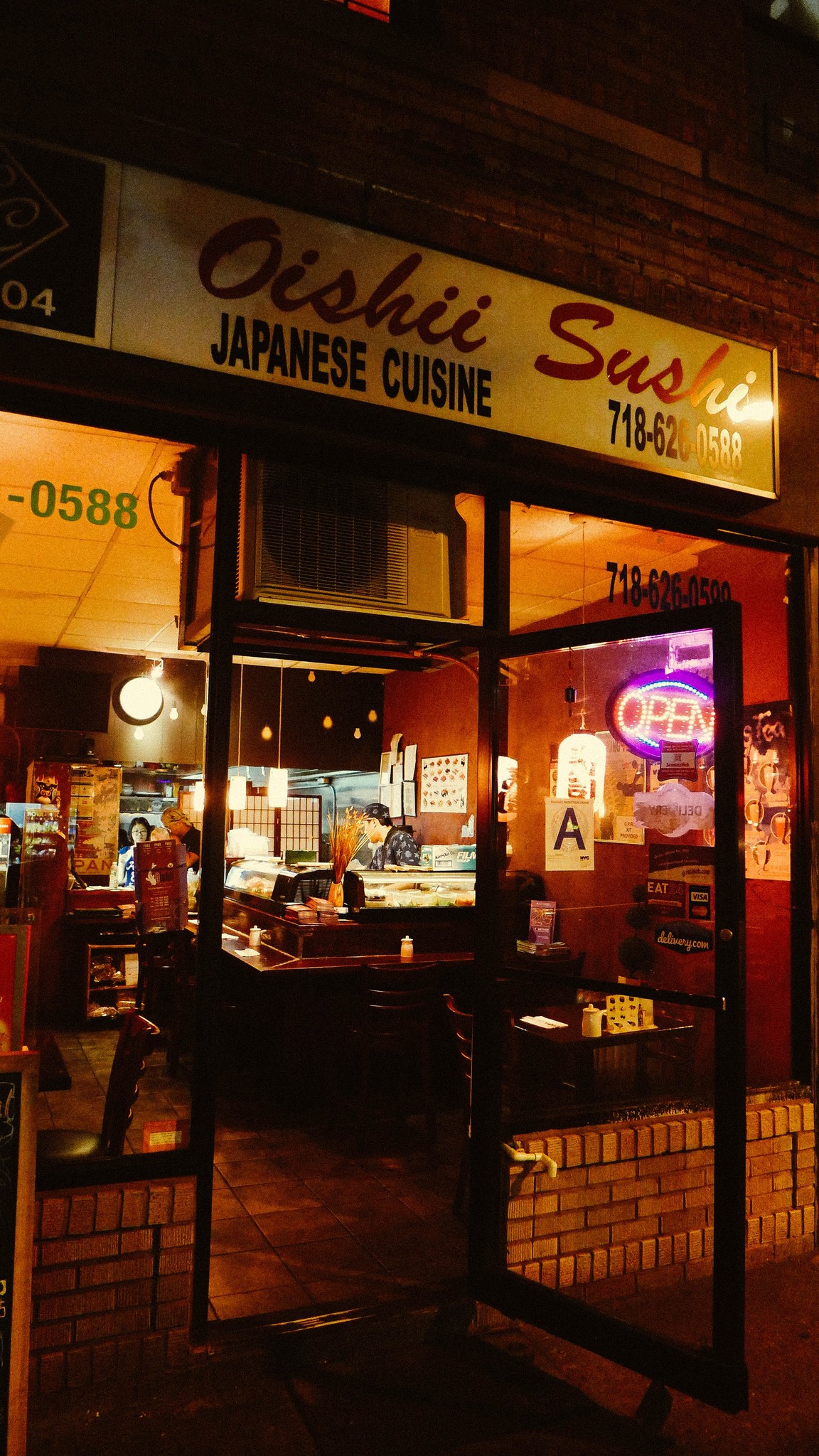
[227,655,249,809]
[109,653,166,728]
[261,655,381,745]
[555,514,610,822]
[192,650,210,815]
[266,657,291,809]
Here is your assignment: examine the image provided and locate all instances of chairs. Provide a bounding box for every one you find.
[442,988,515,1135]
[335,954,446,1131]
[509,943,586,1005]
[35,1009,164,1164]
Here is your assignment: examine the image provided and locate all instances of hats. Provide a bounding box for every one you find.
[361,803,390,820]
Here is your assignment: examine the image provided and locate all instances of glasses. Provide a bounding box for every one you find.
[130,830,147,835]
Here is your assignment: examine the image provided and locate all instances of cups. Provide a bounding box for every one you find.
[582,1004,607,1037]
[709,757,787,873]
[249,925,267,946]
[400,936,414,957]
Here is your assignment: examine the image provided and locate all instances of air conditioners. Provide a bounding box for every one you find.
[158,445,450,671]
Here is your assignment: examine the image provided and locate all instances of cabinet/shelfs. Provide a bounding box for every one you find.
[79,938,147,1027]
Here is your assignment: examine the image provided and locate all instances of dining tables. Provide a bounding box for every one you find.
[21,1021,75,1094]
[516,996,697,1099]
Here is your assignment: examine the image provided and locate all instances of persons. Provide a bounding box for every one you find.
[616,782,644,797]
[600,812,615,840]
[631,760,644,784]
[159,806,201,875]
[353,802,422,891]
[0,803,21,844]
[117,828,130,852]
[116,817,152,888]
